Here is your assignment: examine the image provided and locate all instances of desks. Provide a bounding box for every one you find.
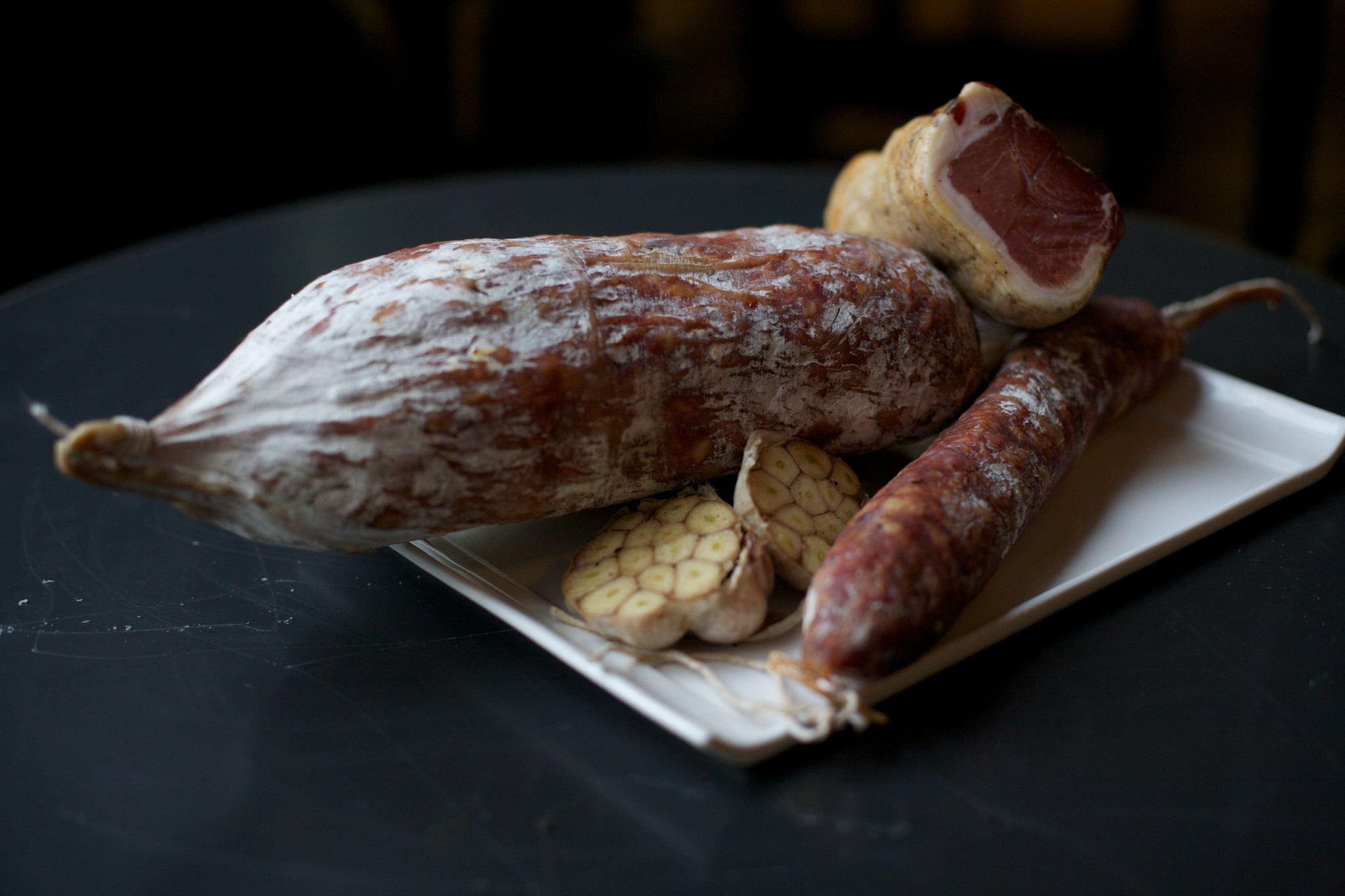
[2,163,1345,896]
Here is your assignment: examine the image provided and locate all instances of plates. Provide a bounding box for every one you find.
[389,361,1345,767]
